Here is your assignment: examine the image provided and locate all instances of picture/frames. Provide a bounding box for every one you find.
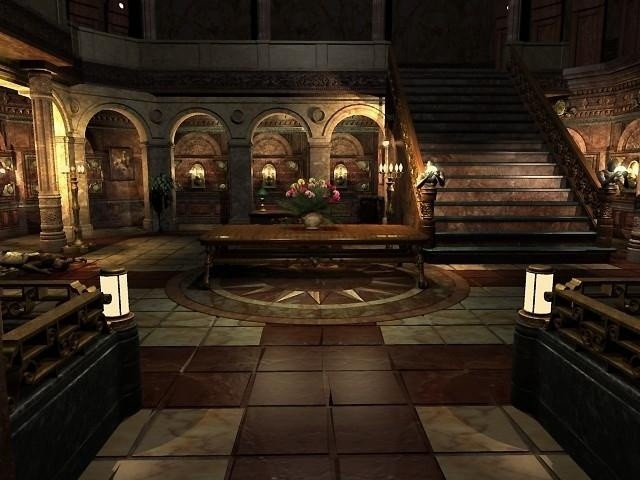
[0,150,39,204]
[85,144,135,199]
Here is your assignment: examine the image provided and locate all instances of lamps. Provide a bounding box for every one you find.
[378,162,404,223]
[522,266,554,318]
[97,264,130,320]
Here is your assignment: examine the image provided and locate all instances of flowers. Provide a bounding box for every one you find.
[275,174,343,214]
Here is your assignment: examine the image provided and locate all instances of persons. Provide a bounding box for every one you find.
[596,157,629,189]
[415,160,445,189]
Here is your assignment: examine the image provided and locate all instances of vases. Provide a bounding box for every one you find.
[302,213,326,230]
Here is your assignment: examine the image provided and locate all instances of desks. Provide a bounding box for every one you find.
[197,224,433,291]
